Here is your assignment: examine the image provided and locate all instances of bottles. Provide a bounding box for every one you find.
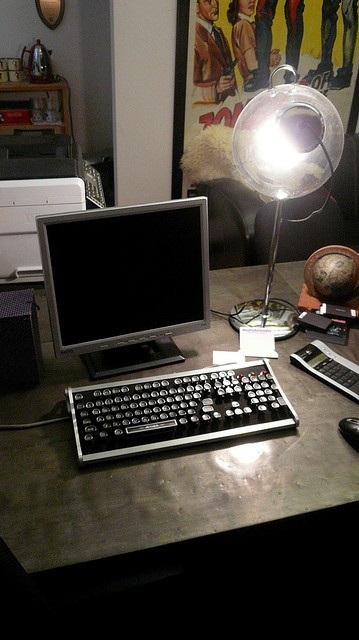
[8,58,21,70]
[8,71,20,82]
[0,71,8,81]
[0,58,8,70]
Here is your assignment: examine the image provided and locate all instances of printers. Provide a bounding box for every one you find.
[0,133,106,287]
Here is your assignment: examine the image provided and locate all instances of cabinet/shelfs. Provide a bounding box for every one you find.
[0,75,72,135]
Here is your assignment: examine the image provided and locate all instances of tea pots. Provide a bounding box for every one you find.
[21,39,54,84]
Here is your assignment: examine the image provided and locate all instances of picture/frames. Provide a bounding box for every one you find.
[35,0,65,30]
[171,0,359,271]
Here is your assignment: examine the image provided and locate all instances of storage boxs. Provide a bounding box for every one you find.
[0,100,31,125]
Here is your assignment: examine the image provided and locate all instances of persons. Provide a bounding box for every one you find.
[245,0,306,93]
[191,0,234,104]
[301,1,358,91]
[226,0,280,100]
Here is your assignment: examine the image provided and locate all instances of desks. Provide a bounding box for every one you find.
[0,260,359,573]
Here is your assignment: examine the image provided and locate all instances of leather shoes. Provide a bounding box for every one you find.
[244,69,270,91]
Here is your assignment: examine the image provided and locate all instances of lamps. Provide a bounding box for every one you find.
[229,65,345,343]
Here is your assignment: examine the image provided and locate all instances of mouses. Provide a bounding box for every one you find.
[339,418,359,452]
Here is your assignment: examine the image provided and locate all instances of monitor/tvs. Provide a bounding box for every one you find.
[35,196,211,382]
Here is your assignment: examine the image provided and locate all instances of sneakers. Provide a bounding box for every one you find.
[302,63,333,83]
[328,67,352,90]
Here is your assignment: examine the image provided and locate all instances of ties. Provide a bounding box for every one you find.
[212,25,227,62]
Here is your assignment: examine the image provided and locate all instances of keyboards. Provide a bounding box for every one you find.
[64,358,299,465]
